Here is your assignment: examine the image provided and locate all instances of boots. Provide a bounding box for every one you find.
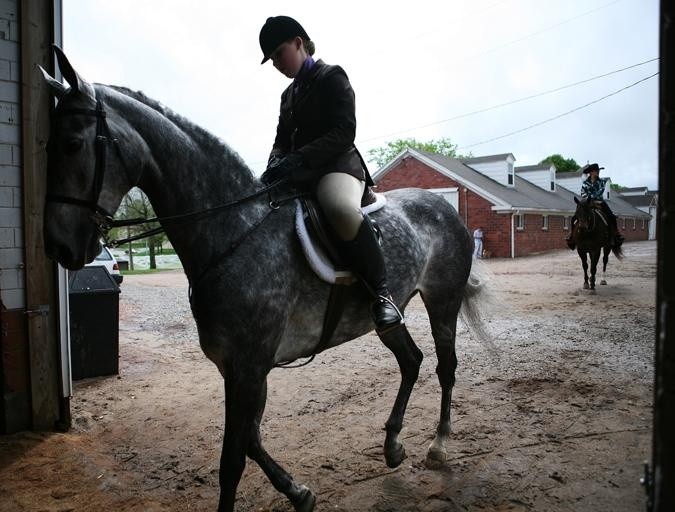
[343,212,402,332]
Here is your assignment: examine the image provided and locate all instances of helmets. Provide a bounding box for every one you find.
[259,15,309,64]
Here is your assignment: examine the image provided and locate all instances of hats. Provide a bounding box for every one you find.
[583,164,605,173]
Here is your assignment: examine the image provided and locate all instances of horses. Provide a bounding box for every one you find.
[572,194,626,295]
[35,42,504,512]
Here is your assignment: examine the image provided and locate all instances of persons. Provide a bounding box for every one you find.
[473,227,484,258]
[566,163,625,241]
[260,16,402,331]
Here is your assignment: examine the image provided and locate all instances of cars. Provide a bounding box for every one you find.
[83,243,124,286]
[108,248,131,273]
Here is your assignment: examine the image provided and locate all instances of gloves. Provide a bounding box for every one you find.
[260,154,301,184]
